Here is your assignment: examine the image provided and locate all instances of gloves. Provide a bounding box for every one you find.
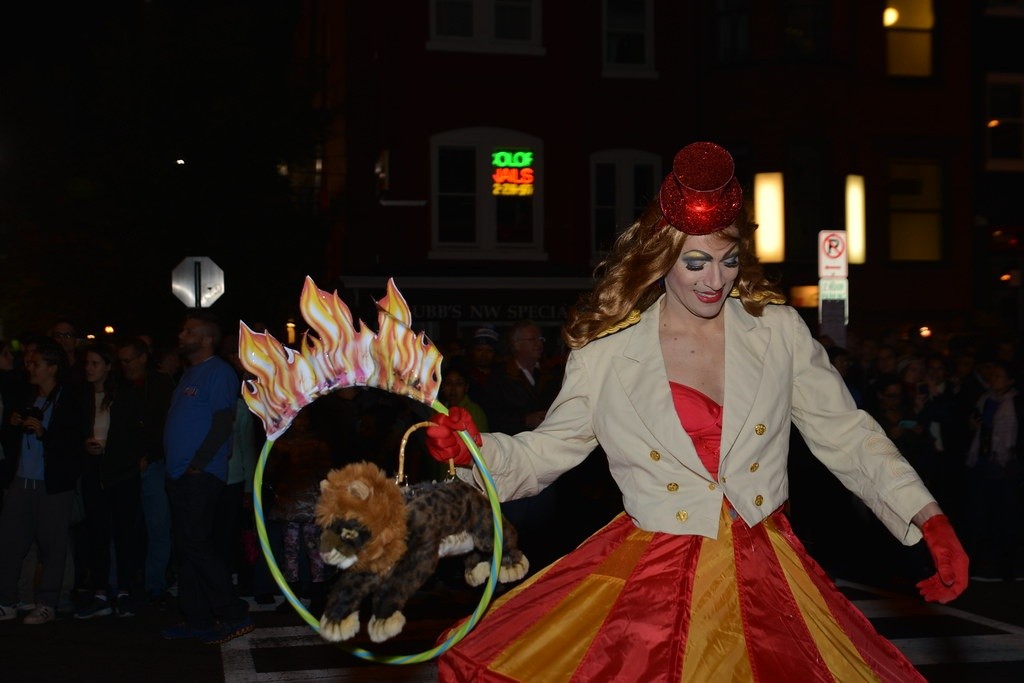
[426,406,482,467]
[915,515,970,604]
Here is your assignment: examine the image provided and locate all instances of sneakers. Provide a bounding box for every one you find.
[160,620,206,639]
[23,604,54,624]
[74,595,112,619]
[0,605,17,619]
[115,595,135,618]
[201,615,255,644]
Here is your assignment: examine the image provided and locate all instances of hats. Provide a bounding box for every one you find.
[473,328,500,346]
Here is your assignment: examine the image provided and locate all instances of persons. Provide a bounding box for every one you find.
[817,325,1024,583]
[428,140,969,683]
[0,310,563,645]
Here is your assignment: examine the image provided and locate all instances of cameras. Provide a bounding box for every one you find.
[19,407,44,435]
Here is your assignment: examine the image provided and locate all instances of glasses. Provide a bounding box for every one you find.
[121,353,141,363]
[55,333,78,338]
[518,336,546,343]
